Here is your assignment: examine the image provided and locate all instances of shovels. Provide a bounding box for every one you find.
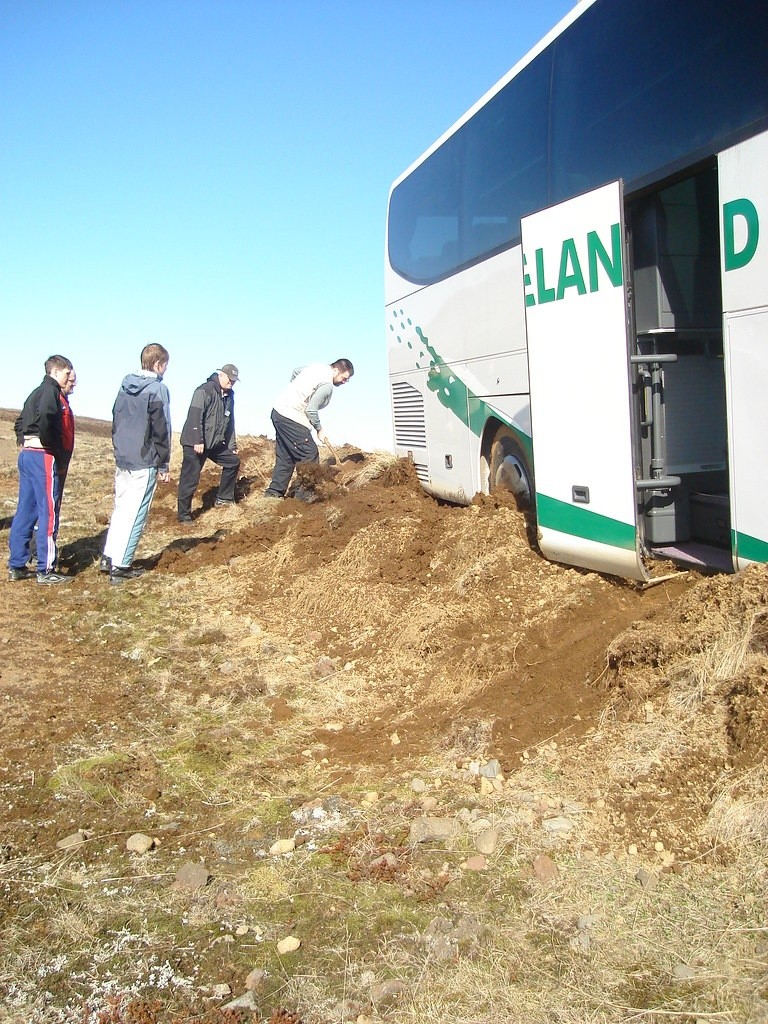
[316,427,354,475]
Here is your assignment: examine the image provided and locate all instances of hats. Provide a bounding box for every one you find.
[216,364,240,382]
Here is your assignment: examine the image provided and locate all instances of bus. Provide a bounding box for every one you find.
[383,1,767,591]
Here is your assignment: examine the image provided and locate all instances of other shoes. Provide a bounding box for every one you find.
[215,498,236,508]
[179,519,196,527]
[99,554,112,573]
[265,491,285,498]
[110,565,143,582]
[301,492,320,504]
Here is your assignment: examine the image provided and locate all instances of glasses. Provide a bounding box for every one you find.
[338,371,349,383]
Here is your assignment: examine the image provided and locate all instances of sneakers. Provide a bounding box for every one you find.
[36,572,75,586]
[8,566,38,582]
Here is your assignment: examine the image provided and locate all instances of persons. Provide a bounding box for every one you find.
[29,368,76,562]
[177,363,243,527]
[99,342,172,584]
[263,359,354,501]
[8,355,76,585]
